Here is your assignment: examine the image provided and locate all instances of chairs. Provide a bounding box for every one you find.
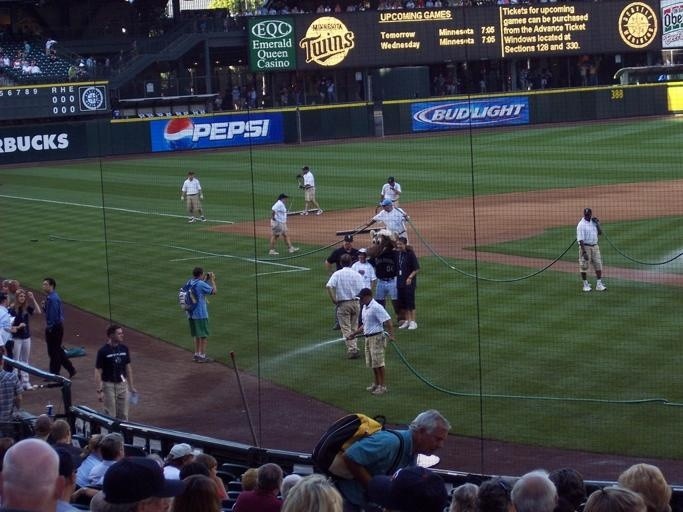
[0,42,91,83]
[0,415,284,512]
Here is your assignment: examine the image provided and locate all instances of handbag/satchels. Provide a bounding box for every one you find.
[311,413,404,477]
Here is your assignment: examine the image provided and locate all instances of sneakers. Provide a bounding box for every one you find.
[333,321,341,330]
[583,283,606,291]
[269,249,279,255]
[397,317,418,330]
[191,353,208,364]
[344,349,360,360]
[22,382,34,389]
[188,216,207,223]
[300,209,323,216]
[366,383,387,394]
[43,368,75,382]
[289,245,299,253]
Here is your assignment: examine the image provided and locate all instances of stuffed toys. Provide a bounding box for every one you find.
[361,228,400,315]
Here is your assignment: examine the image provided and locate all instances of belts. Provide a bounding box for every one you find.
[365,332,382,338]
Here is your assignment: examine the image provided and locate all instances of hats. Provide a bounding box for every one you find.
[302,166,309,170]
[356,289,372,297]
[344,234,354,242]
[170,443,203,460]
[102,456,187,505]
[382,199,393,207]
[279,194,288,200]
[584,208,591,214]
[358,247,368,254]
[368,466,449,512]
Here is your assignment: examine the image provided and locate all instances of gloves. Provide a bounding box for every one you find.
[582,251,588,260]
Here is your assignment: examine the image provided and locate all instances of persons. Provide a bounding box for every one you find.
[634,59,681,83]
[181,171,207,223]
[67,56,96,83]
[46,38,58,61]
[431,71,460,96]
[24,41,31,54]
[0,279,682,512]
[312,75,336,106]
[381,176,401,209]
[576,59,600,87]
[479,77,488,94]
[104,56,110,66]
[187,267,217,363]
[199,0,557,34]
[324,236,361,329]
[115,37,140,73]
[505,66,552,92]
[0,49,44,77]
[299,166,324,216]
[326,256,371,360]
[214,86,256,110]
[351,249,376,289]
[268,192,299,257]
[364,201,411,244]
[577,206,608,293]
[395,238,425,331]
[278,84,303,106]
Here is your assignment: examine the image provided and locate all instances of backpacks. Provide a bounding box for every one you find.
[178,279,201,312]
